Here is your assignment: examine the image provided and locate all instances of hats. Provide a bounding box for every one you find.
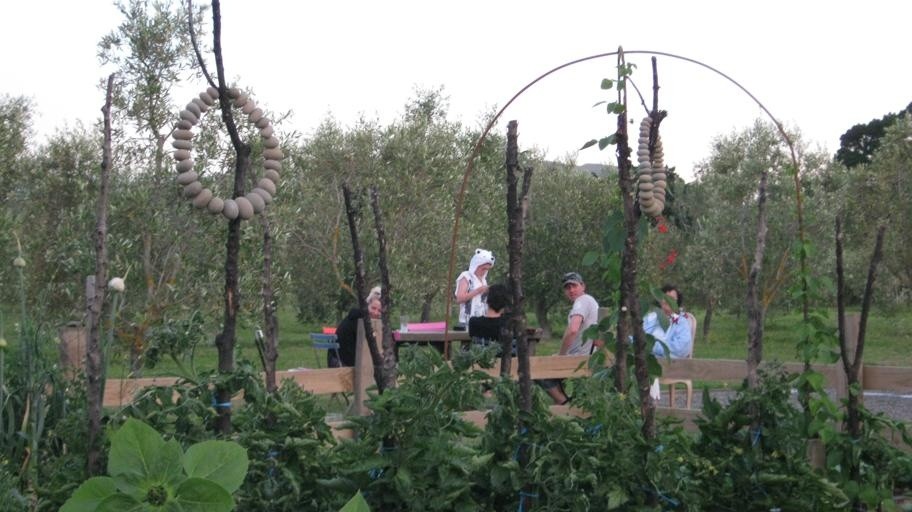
[562,272,583,289]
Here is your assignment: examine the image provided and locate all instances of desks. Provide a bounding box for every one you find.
[390,322,543,367]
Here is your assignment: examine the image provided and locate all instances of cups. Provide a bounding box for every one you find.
[399,314,409,337]
[453,309,469,331]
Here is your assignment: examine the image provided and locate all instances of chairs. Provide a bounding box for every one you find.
[652,310,699,410]
[555,323,606,405]
[308,330,358,410]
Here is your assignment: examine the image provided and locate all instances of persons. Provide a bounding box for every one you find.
[628,286,692,407]
[453,248,495,350]
[468,283,517,358]
[534,272,599,403]
[329,296,382,369]
